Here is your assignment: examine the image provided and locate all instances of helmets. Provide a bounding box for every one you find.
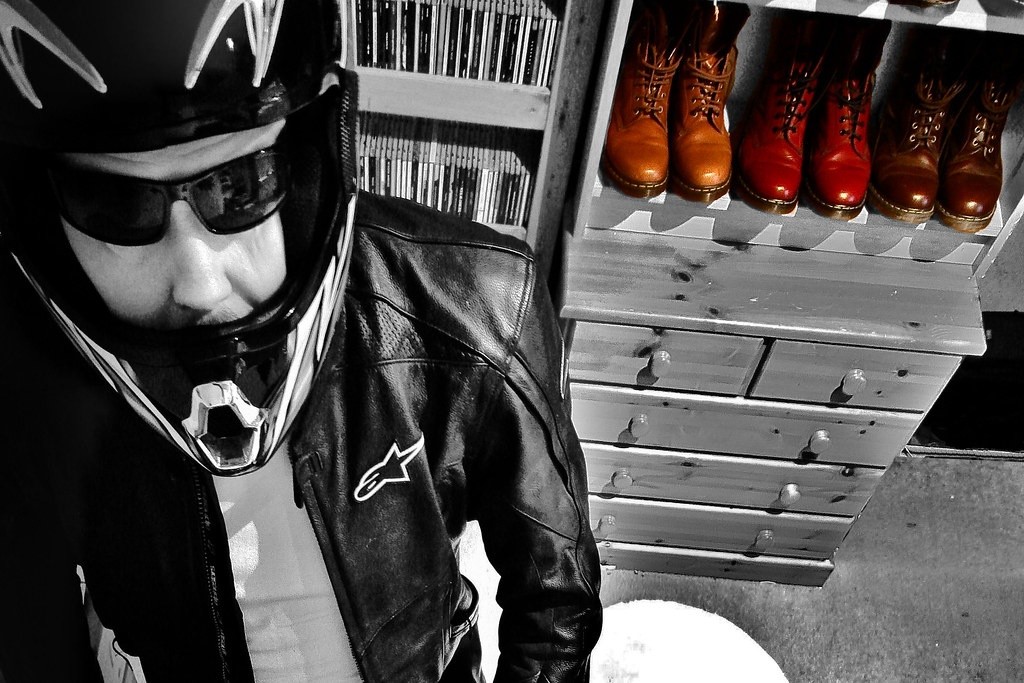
[0,1,359,476]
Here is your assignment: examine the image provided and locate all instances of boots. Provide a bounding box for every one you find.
[871,28,968,221]
[673,2,750,201]
[933,33,1024,231]
[737,18,830,208]
[599,0,691,198]
[802,21,892,213]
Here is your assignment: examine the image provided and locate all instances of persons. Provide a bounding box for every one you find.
[0,0,603,683]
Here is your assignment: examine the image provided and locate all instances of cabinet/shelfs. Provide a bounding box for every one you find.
[557,205,987,589]
[345,0,569,251]
[570,0,1024,280]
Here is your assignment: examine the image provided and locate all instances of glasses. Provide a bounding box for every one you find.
[54,140,290,246]
